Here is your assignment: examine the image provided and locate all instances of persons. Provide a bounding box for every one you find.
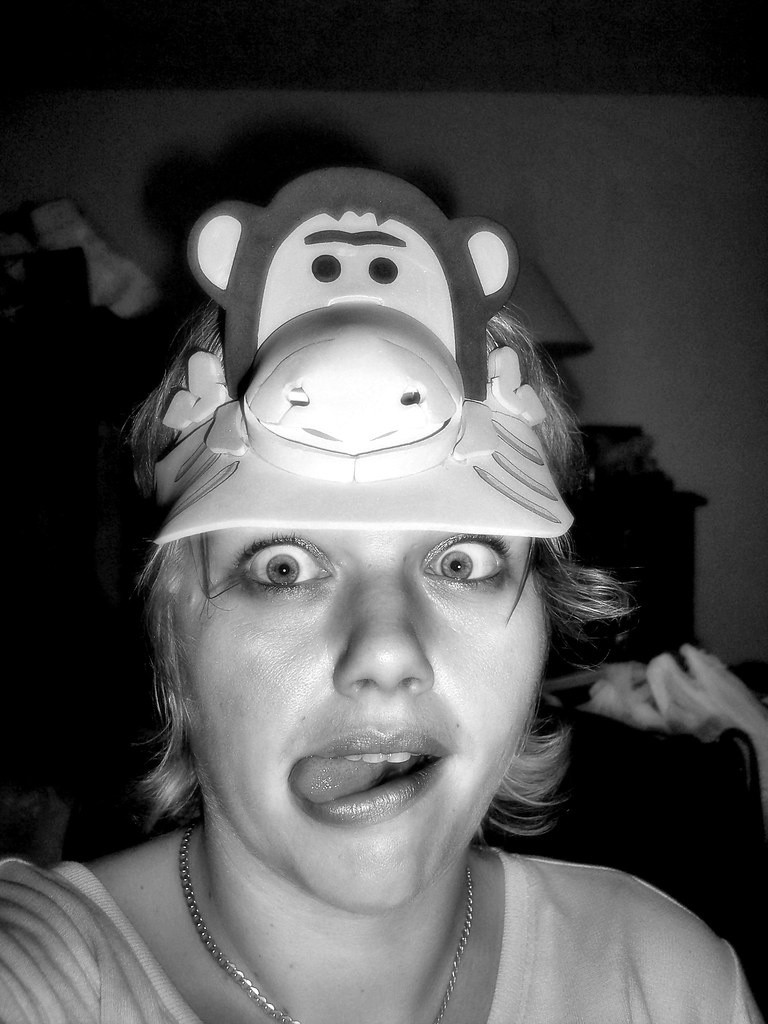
[0,168,768,1024]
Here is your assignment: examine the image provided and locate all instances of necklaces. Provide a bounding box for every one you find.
[180,821,472,1024]
